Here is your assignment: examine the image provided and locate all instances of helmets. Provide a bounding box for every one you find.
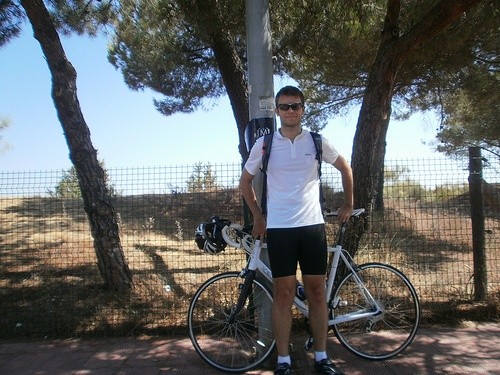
[194,217,231,256]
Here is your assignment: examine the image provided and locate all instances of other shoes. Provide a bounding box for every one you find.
[274,358,296,375]
[314,355,346,375]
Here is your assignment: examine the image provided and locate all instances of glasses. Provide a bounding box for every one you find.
[276,103,304,111]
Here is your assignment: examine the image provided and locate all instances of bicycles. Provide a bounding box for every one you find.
[186,208,421,374]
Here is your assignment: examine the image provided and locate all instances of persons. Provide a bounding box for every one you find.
[240,86,353,375]
